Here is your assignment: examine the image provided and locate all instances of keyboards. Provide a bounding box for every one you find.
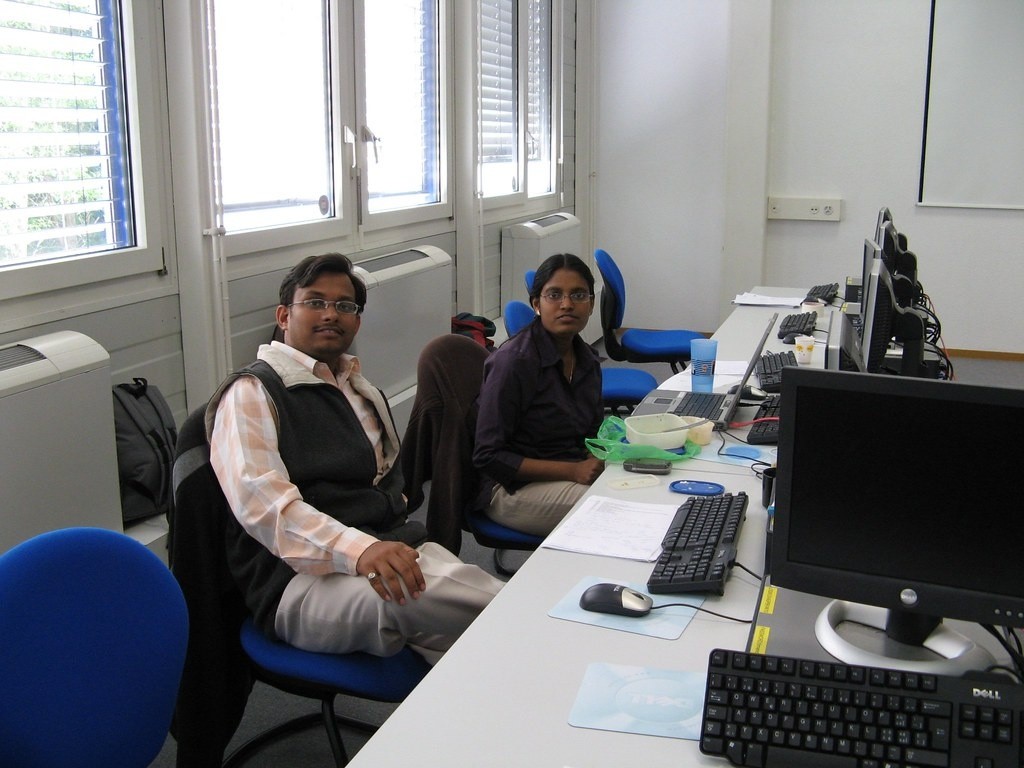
[747,395,781,445]
[754,351,798,393]
[778,311,817,339]
[807,283,839,305]
[647,491,749,598]
[699,649,1024,768]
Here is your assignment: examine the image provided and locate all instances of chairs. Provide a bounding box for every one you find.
[0,527,190,768]
[503,269,658,421]
[594,248,707,375]
[465,510,545,577]
[170,402,432,768]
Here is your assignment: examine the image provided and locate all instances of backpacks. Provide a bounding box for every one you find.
[111,378,179,524]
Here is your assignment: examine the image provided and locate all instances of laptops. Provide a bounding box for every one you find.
[631,313,779,431]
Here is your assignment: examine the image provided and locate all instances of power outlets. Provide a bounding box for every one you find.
[767,196,840,222]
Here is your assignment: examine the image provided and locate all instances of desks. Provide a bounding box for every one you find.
[345,285,1024,768]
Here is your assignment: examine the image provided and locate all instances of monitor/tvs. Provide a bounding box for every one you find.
[770,207,1024,677]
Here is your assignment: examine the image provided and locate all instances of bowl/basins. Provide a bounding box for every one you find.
[623,413,686,452]
[681,415,714,446]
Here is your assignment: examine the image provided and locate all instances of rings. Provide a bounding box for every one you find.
[367,572,377,580]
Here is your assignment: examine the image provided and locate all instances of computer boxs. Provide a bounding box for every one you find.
[746,577,1017,678]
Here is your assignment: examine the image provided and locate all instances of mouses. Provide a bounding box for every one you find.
[800,296,828,307]
[726,386,767,400]
[579,584,654,617]
[782,332,808,344]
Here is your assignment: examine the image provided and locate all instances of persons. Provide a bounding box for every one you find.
[204,252,506,662]
[474,255,604,537]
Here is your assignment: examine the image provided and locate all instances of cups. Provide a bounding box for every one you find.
[690,338,717,393]
[795,337,815,363]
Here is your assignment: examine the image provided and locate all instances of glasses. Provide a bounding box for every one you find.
[283,298,361,314]
[539,292,594,303]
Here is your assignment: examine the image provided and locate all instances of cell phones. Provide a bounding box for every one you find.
[622,458,673,475]
